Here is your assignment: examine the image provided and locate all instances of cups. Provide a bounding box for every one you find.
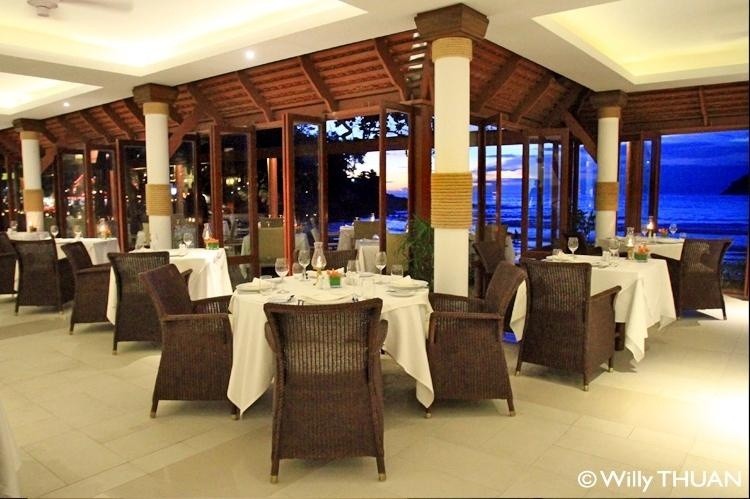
[10,221,18,229]
[625,246,634,260]
[260,275,272,295]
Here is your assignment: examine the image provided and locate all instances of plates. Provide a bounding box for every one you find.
[388,289,416,298]
[389,279,430,289]
[236,282,274,292]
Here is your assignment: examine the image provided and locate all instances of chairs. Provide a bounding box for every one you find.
[2,210,734,484]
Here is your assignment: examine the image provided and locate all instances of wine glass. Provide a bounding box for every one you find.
[72,225,83,242]
[50,224,59,241]
[184,233,194,254]
[550,235,621,268]
[625,221,678,243]
[273,240,404,303]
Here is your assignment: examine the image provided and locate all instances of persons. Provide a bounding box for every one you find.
[184,182,209,223]
